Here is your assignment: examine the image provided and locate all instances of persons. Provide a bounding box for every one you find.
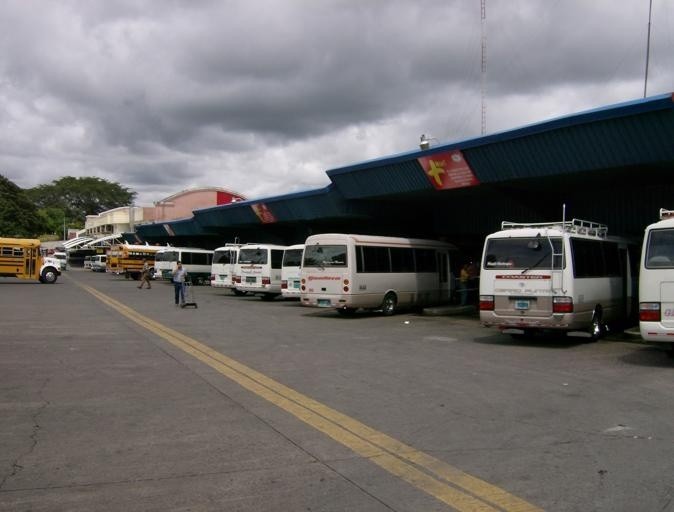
[136,258,151,290]
[171,261,188,307]
[459,261,481,307]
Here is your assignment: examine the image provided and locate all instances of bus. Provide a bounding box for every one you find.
[300,232,466,317]
[479,204,643,342]
[154,247,215,285]
[638,206,674,342]
[209,237,307,299]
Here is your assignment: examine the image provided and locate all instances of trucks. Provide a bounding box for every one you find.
[106,243,177,280]
[0,237,62,285]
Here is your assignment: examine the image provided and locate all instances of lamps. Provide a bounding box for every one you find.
[419,134,440,151]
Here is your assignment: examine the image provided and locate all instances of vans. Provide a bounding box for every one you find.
[53,251,67,269]
[83,254,107,272]
